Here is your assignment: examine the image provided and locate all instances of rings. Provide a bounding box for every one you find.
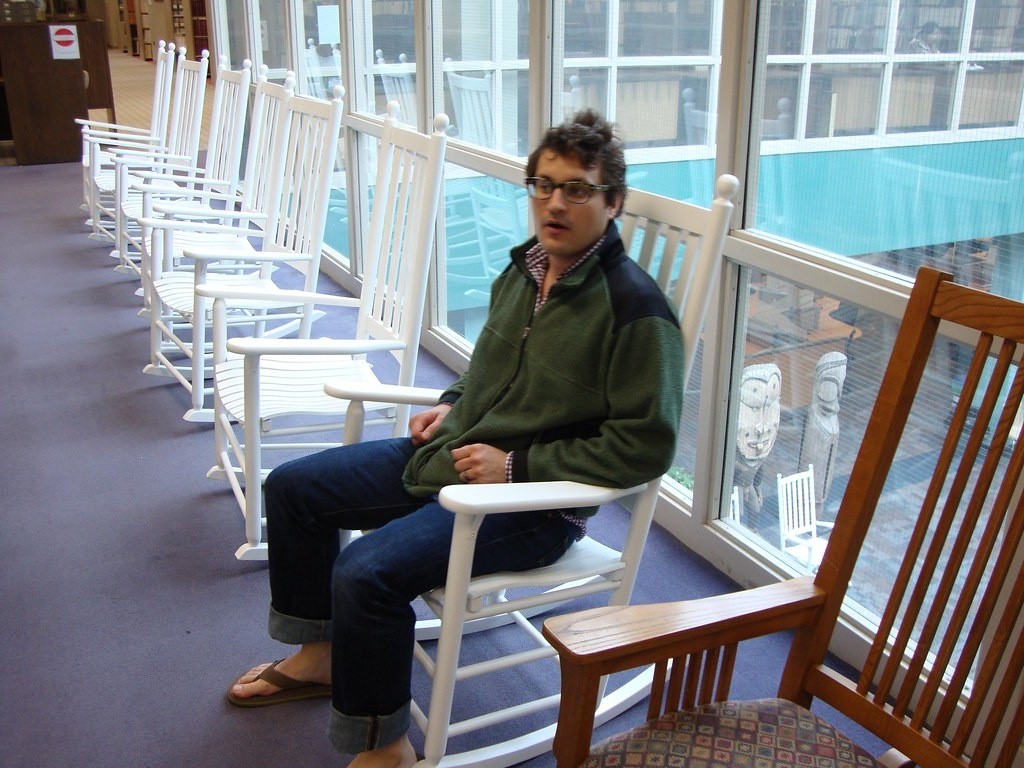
[463,471,471,480]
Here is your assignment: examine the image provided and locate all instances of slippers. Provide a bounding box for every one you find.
[226,658,332,708]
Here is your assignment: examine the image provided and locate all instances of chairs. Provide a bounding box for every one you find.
[75,40,1024,767]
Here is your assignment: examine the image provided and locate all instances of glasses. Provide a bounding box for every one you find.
[523,177,615,205]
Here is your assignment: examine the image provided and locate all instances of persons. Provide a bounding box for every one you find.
[909,21,941,54]
[226,107,686,768]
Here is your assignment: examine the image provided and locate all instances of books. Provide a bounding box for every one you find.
[118,0,211,79]
[827,0,1022,61]
